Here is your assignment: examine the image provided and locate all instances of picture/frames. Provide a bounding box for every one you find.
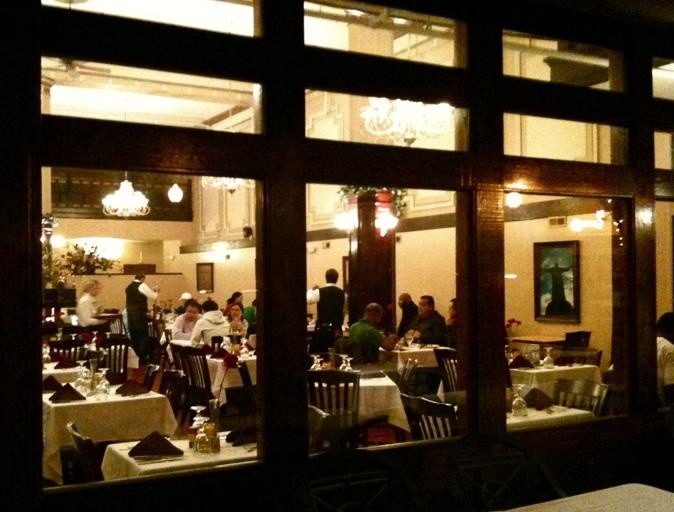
[534,240,580,324]
[196,263,213,293]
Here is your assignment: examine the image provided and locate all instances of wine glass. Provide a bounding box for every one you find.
[404,335,414,349]
[190,402,213,455]
[220,335,248,357]
[541,345,555,370]
[310,352,354,371]
[72,359,110,398]
[511,382,528,417]
[54,330,111,341]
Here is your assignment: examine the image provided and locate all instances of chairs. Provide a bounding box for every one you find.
[307,370,367,448]
[553,379,612,416]
[308,404,332,453]
[158,369,191,419]
[400,393,458,441]
[66,423,104,481]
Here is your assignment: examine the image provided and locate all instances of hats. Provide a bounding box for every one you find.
[178,291,194,300]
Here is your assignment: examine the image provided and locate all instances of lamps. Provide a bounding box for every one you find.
[101,171,151,220]
[359,34,455,147]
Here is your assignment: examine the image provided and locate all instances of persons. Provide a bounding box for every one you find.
[159,289,458,409]
[75,278,104,332]
[656,312,673,411]
[123,271,158,367]
[307,269,345,351]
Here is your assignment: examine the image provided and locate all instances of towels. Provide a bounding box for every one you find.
[129,431,184,457]
[226,411,258,446]
[49,383,86,400]
